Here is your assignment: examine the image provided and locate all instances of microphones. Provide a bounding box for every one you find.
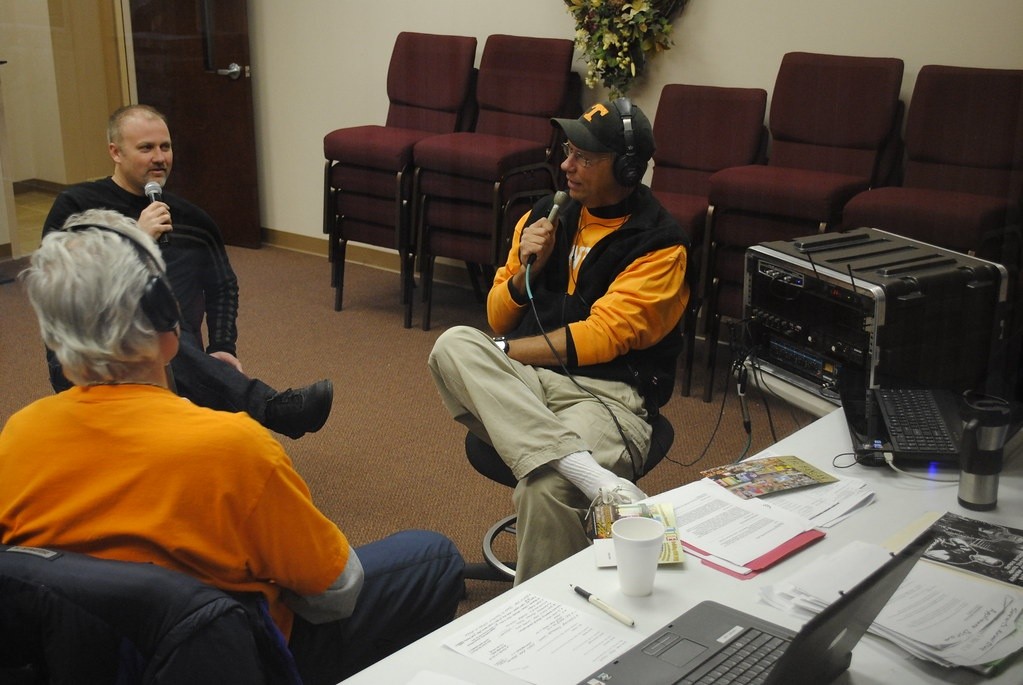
[528,190,568,264]
[143,181,170,247]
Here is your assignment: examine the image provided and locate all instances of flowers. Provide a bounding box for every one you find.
[562,0,692,103]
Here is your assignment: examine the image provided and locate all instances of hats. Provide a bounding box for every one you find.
[548,99,654,162]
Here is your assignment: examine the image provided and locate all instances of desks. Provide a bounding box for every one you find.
[331,406,1023,685]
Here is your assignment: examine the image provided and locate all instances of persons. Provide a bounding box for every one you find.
[45,103,333,440]
[427,97,696,590]
[923,537,1004,568]
[0,207,466,685]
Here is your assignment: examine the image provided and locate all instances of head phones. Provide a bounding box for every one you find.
[610,96,648,187]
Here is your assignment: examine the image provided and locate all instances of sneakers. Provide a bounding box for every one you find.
[258,379,333,439]
[583,477,651,520]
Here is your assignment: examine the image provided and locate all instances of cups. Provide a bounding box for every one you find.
[611,517,665,598]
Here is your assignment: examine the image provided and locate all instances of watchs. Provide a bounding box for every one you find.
[492,336,510,355]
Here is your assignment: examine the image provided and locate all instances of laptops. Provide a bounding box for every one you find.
[577,530,937,685]
[841,327,1023,464]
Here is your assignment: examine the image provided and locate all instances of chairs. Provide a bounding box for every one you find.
[0,541,272,685]
[322,31,1023,579]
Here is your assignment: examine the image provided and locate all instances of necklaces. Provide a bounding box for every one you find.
[86,380,164,388]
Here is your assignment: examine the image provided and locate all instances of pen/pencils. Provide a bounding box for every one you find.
[570,584,634,627]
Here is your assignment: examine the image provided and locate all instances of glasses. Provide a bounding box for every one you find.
[558,142,617,169]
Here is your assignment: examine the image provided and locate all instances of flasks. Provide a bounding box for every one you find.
[957,389,1011,511]
